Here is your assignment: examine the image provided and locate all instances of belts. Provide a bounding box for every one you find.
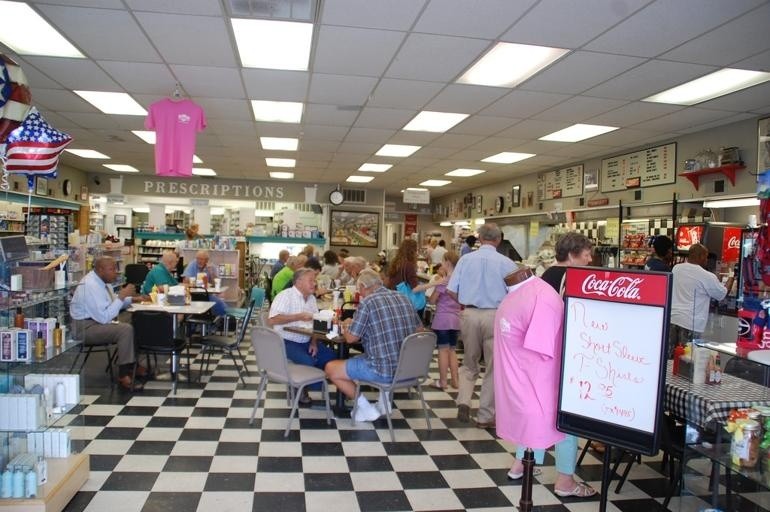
[464,304,478,309]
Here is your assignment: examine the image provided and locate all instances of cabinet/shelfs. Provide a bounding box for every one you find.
[1,233,91,506]
[137,245,176,271]
[68,240,127,294]
[22,206,78,255]
[87,212,104,234]
[248,238,331,284]
[236,240,246,290]
[135,232,186,241]
[182,248,240,308]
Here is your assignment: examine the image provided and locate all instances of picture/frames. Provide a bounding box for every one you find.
[443,207,448,217]
[543,162,585,201]
[476,195,483,214]
[114,214,126,225]
[329,209,380,248]
[755,117,770,184]
[512,184,522,207]
[600,141,677,194]
[35,176,49,196]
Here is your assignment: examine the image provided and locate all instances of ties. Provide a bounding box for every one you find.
[197,263,207,273]
[105,284,120,325]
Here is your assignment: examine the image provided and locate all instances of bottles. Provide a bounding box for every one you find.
[672,344,692,375]
[0,467,13,499]
[55,382,65,406]
[705,355,722,385]
[53,322,62,347]
[35,331,45,359]
[14,306,24,328]
[25,469,38,498]
[35,456,47,485]
[12,470,24,500]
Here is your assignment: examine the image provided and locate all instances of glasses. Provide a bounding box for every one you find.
[298,278,318,283]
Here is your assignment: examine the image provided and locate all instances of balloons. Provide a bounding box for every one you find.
[0,50,30,156]
[6,106,74,189]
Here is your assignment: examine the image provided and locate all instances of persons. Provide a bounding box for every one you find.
[321,251,343,280]
[447,223,519,428]
[377,250,387,266]
[183,250,228,316]
[352,256,382,293]
[304,246,321,272]
[497,231,524,262]
[644,236,675,271]
[267,268,337,404]
[324,273,424,422]
[424,263,445,299]
[668,244,734,360]
[427,241,436,264]
[270,250,289,278]
[389,239,448,318]
[70,256,148,390]
[142,250,177,297]
[430,240,447,275]
[271,256,297,301]
[282,257,322,290]
[340,248,348,254]
[338,254,352,281]
[508,233,597,497]
[337,256,356,286]
[430,252,459,389]
[493,266,565,512]
[460,236,475,256]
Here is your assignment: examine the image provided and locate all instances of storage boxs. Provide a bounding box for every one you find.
[12,268,54,291]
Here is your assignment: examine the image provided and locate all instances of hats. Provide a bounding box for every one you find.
[654,236,673,257]
[377,250,386,258]
[466,236,476,243]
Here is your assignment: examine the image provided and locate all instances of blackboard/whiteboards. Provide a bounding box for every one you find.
[556,265,672,457]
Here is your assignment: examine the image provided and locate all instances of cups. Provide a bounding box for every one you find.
[334,279,341,288]
[157,294,166,306]
[333,290,340,302]
[214,279,222,289]
[10,275,22,291]
[748,215,757,227]
[54,271,65,290]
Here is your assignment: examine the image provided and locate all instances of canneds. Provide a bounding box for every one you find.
[719,405,769,467]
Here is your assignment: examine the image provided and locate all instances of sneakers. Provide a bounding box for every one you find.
[351,395,383,424]
[371,400,392,417]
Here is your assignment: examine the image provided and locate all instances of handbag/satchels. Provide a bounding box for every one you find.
[396,261,427,312]
[340,301,360,321]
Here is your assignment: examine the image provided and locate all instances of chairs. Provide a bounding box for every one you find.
[351,330,438,447]
[188,287,210,300]
[660,425,716,488]
[125,263,149,294]
[130,310,191,393]
[71,339,116,383]
[204,313,238,376]
[223,287,266,340]
[257,311,293,407]
[262,269,272,289]
[197,296,256,389]
[701,356,770,462]
[249,324,331,438]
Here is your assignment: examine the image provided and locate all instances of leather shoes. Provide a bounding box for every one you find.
[478,421,497,430]
[457,403,470,425]
[117,376,143,392]
[129,367,150,380]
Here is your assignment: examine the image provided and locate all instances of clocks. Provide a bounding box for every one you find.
[495,196,504,213]
[63,178,72,196]
[329,190,344,206]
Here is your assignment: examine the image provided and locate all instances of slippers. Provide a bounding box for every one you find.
[447,379,459,389]
[552,480,599,499]
[429,378,449,390]
[507,466,544,481]
